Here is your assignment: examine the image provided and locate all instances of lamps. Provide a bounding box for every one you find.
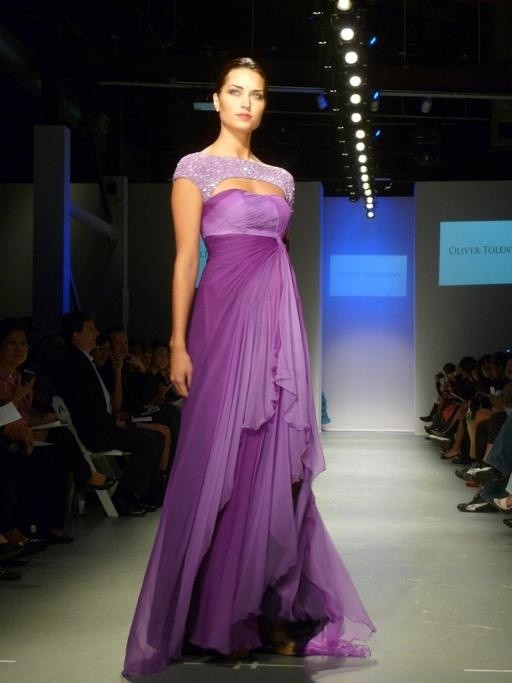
[311,0,384,219]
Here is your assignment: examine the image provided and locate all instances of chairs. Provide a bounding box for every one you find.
[54,396,153,517]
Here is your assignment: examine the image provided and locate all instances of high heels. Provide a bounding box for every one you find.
[224,613,305,660]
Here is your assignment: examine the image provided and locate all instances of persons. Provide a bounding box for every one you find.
[0,307,186,581]
[412,343,511,534]
[118,53,379,678]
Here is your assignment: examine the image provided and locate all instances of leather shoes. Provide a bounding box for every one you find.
[419,413,511,531]
[0,479,171,585]
